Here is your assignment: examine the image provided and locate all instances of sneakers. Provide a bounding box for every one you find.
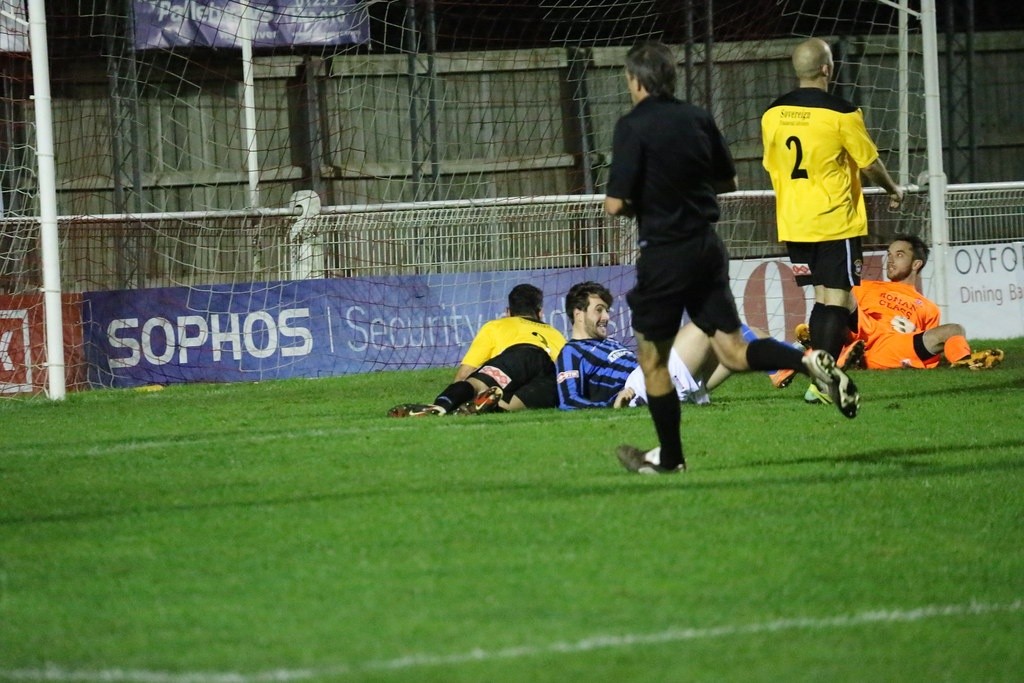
[450,386,503,415]
[805,348,861,419]
[794,322,811,351]
[835,340,865,373]
[803,378,835,407]
[949,347,1005,372]
[767,348,814,389]
[612,443,687,476]
[387,402,446,418]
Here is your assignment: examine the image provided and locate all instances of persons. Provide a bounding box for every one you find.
[760,38,903,405]
[794,234,1004,372]
[388,283,568,418]
[556,281,797,411]
[604,40,861,473]
